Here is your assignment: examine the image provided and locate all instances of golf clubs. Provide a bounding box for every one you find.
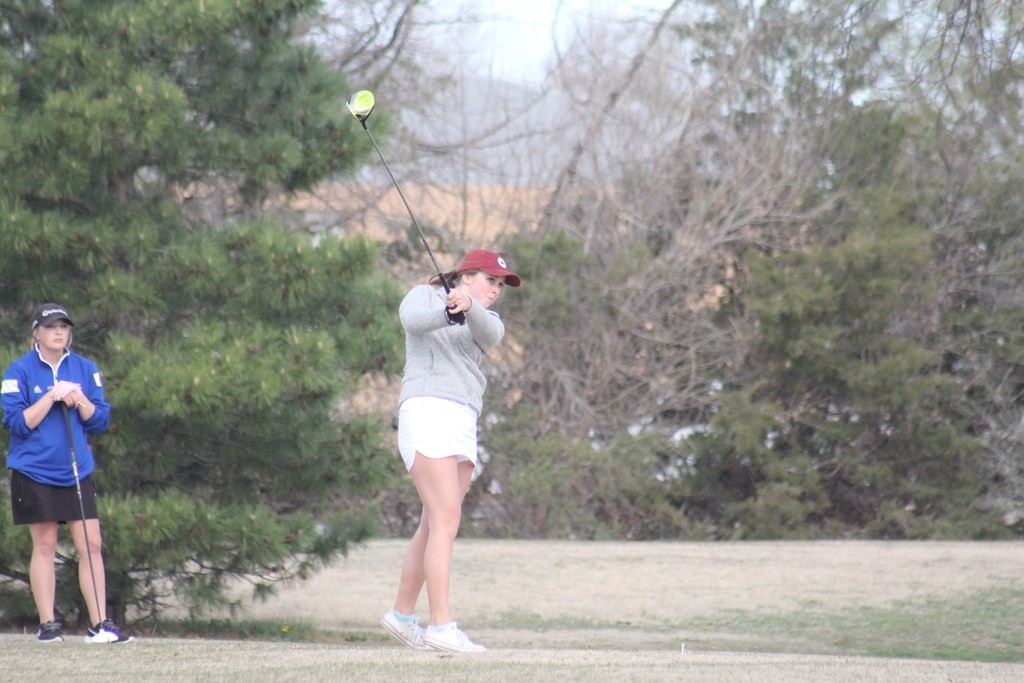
[61,401,119,643]
[345,90,466,326]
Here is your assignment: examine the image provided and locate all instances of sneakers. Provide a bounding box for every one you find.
[84,618,133,644]
[379,608,434,650]
[36,619,65,644]
[424,622,486,652]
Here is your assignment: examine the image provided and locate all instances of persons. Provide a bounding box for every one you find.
[381,250,522,652]
[0,304,135,644]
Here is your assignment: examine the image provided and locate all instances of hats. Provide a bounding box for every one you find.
[31,303,74,331]
[456,250,521,287]
